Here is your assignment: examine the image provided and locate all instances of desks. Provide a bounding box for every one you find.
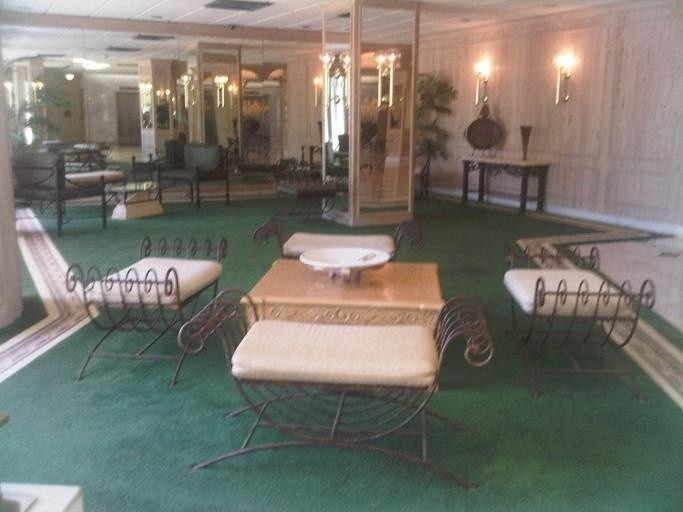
[236,258,446,329]
[460,155,549,213]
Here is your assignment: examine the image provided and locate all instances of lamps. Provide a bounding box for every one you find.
[324,53,351,108]
[374,51,404,111]
[474,62,490,105]
[555,50,574,106]
[214,76,228,107]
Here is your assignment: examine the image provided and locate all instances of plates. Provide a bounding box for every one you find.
[297,246,392,282]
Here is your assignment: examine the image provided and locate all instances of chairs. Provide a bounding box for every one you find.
[39,139,113,171]
[414,152,431,200]
[157,142,233,212]
[127,138,184,193]
[6,150,126,238]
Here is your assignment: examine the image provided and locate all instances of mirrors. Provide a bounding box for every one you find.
[139,78,152,128]
[324,7,350,214]
[189,51,198,146]
[201,52,239,161]
[359,3,413,215]
[152,81,171,129]
[0,17,324,206]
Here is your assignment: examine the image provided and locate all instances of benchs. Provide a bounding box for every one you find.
[276,168,336,218]
[326,177,348,208]
[178,287,497,491]
[504,241,655,353]
[253,217,427,266]
[63,237,228,384]
